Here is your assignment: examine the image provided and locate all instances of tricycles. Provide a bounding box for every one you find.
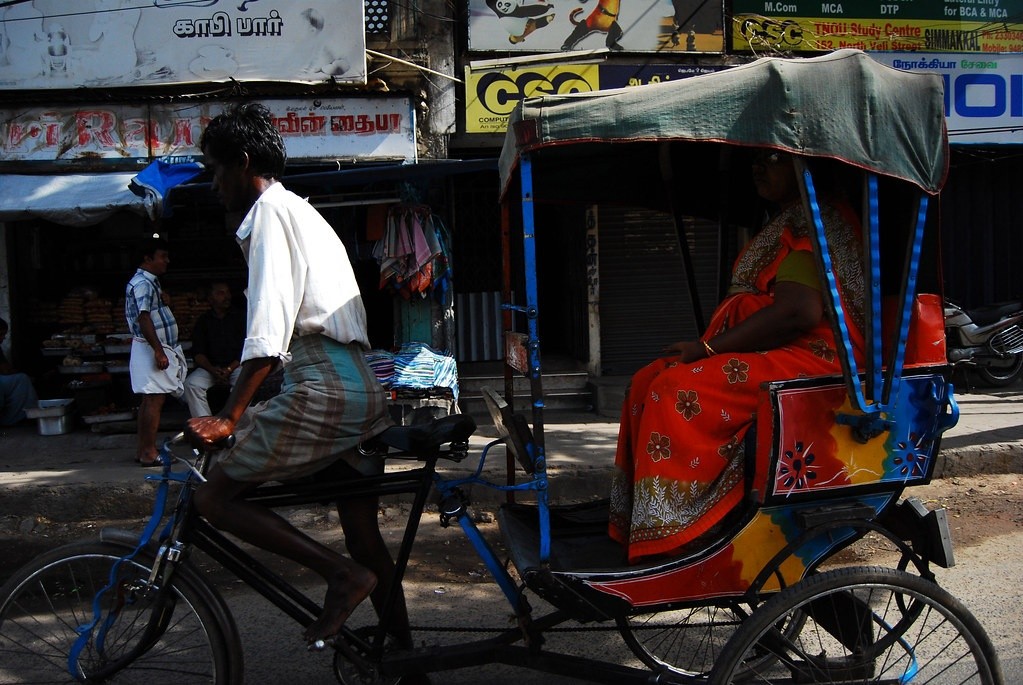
[0,46,1006,684]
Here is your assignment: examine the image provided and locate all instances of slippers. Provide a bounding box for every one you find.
[135,457,141,463]
[142,455,178,467]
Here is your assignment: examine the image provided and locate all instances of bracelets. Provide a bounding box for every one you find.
[702,340,718,358]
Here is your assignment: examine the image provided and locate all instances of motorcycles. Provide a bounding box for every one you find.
[939,296,1023,389]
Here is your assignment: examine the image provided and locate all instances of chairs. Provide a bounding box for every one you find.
[750,292,959,513]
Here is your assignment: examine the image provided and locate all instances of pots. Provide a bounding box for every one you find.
[37,415,72,436]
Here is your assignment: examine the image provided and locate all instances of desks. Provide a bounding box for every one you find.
[389,397,453,427]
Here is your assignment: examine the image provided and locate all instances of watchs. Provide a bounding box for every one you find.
[226,366,233,372]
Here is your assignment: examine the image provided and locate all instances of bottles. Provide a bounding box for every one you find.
[48,24,67,77]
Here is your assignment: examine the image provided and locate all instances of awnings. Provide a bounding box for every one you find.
[0,163,405,214]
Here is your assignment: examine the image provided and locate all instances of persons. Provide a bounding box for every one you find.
[125,241,180,468]
[609,147,883,565]
[0,317,38,426]
[186,103,413,656]
[184,281,245,418]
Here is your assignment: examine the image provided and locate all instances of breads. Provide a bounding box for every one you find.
[54,293,211,328]
